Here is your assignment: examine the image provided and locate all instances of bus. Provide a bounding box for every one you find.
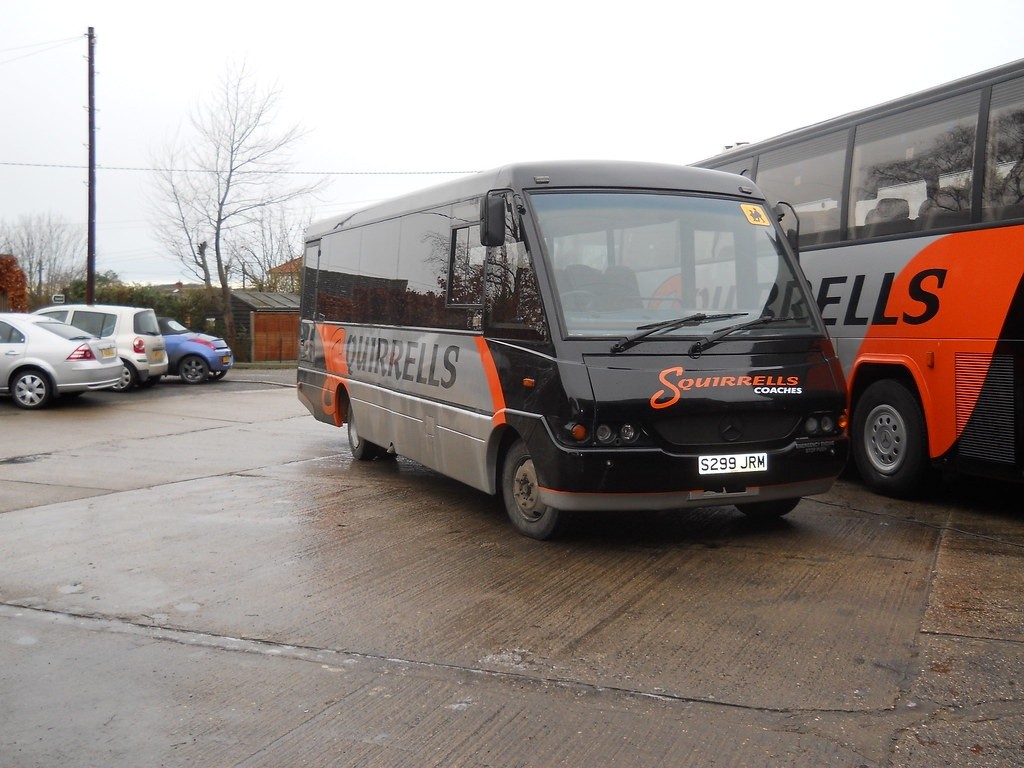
[682,58,1024,501]
[294,158,852,542]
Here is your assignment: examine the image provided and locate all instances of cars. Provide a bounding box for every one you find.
[0,312,125,410]
[149,316,234,385]
[30,304,169,394]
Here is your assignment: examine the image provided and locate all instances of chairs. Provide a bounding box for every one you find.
[781,185,1005,239]
[563,263,601,291]
[593,265,645,317]
